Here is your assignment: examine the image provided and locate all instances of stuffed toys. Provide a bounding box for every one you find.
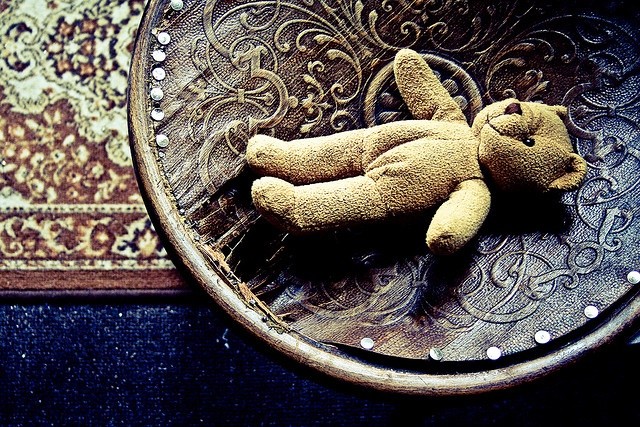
[246,49,587,254]
